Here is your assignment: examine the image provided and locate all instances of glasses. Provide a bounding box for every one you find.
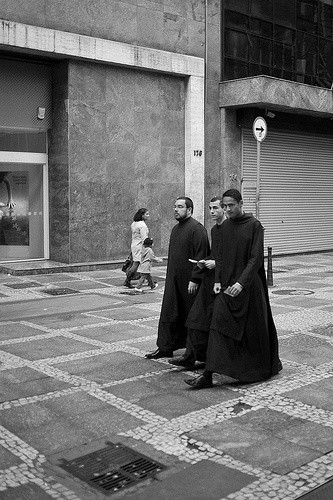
[172,205,187,209]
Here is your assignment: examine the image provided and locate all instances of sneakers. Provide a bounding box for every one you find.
[134,287,143,293]
[151,283,158,290]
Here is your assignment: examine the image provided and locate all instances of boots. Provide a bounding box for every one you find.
[122,271,137,288]
[147,281,157,286]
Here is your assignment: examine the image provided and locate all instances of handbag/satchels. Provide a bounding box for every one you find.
[121,252,143,280]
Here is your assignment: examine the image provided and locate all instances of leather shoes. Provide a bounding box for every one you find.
[144,348,173,359]
[184,374,212,388]
[167,354,195,368]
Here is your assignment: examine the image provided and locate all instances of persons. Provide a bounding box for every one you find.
[184,189,283,388]
[135,237,163,292]
[169,195,229,368]
[145,197,211,359]
[122,208,157,288]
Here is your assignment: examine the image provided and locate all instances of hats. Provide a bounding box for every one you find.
[143,238,153,245]
